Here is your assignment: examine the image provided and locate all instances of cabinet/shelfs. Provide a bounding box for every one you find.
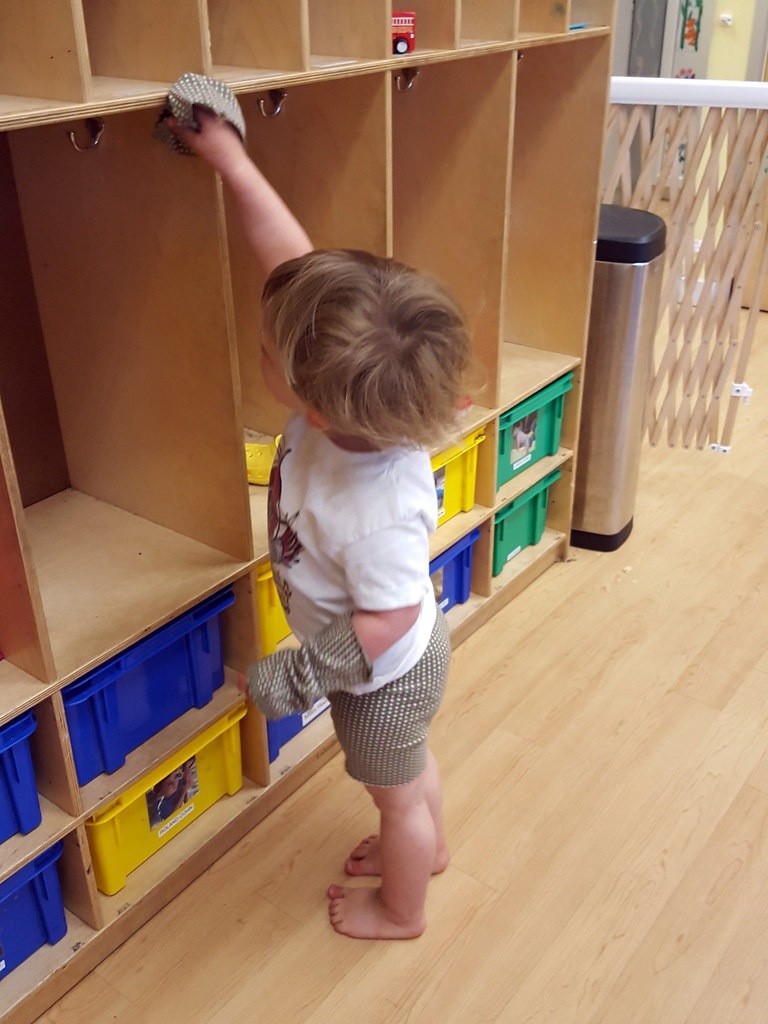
[0,0,618,1024]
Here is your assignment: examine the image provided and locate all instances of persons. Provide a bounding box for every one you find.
[164,74,471,941]
[153,755,199,822]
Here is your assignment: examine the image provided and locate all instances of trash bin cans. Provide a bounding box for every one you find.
[570,202,670,553]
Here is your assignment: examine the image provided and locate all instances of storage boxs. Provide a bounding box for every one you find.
[492,464,562,578]
[496,371,574,494]
[65,582,237,789]
[257,562,294,656]
[0,839,68,981]
[430,423,488,530]
[0,710,42,846]
[429,527,482,614]
[267,695,331,766]
[90,701,248,896]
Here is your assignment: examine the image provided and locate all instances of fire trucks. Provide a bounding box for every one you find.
[392,12,418,54]
